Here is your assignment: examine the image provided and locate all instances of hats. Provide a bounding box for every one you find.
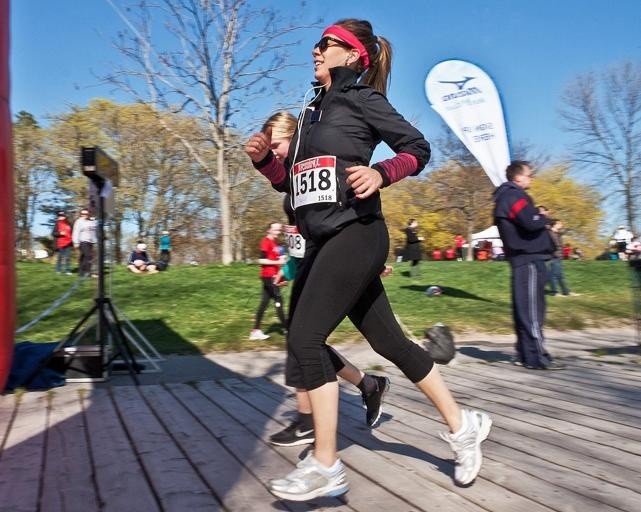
[137,243,148,252]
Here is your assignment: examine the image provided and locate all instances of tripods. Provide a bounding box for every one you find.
[50,229,141,389]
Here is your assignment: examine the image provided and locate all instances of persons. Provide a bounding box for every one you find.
[127,242,159,274]
[52,210,75,277]
[549,219,583,298]
[159,231,173,263]
[268,110,391,450]
[536,205,560,297]
[606,225,641,270]
[71,209,96,277]
[432,233,584,262]
[493,160,567,373]
[249,221,287,341]
[405,218,425,267]
[243,17,493,507]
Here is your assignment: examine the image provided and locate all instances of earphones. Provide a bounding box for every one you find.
[345,53,353,62]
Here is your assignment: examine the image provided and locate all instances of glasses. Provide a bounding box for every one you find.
[312,35,354,51]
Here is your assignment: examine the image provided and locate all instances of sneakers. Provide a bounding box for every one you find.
[437,407,494,487]
[247,328,271,341]
[267,418,318,447]
[360,374,392,429]
[510,353,571,373]
[264,449,351,503]
